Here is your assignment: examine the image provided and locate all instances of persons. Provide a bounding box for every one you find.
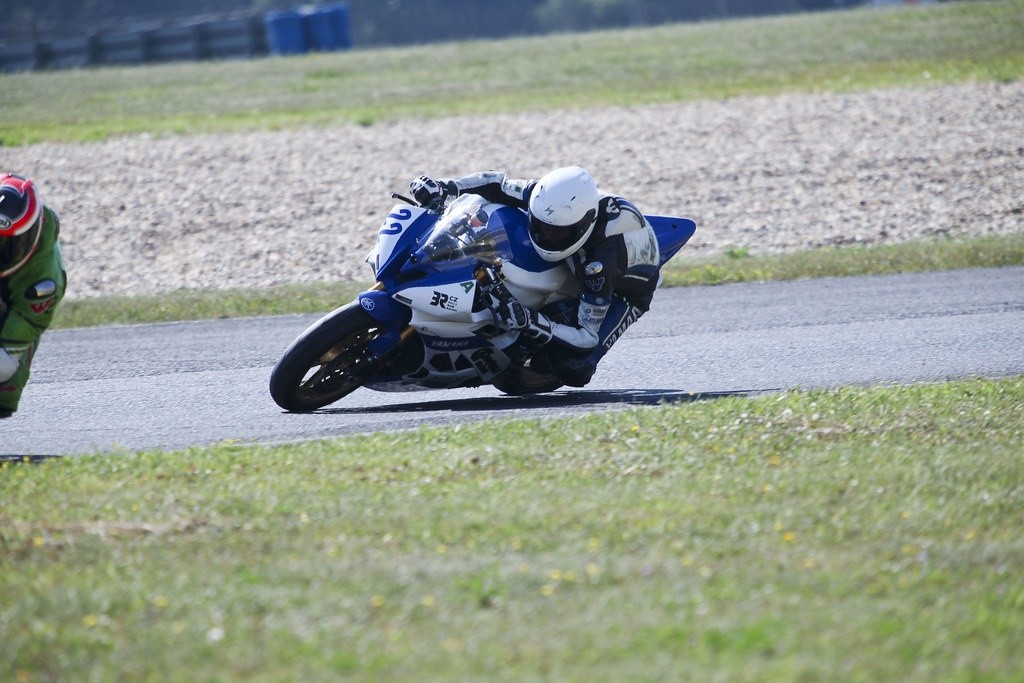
[408,165,664,393]
[1,168,69,423]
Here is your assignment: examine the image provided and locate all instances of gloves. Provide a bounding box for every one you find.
[409,175,459,209]
[500,300,553,346]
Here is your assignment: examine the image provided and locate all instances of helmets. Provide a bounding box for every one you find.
[527,166,599,262]
[0,173,44,278]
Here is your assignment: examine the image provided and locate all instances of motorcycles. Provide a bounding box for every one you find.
[266,189,698,419]
